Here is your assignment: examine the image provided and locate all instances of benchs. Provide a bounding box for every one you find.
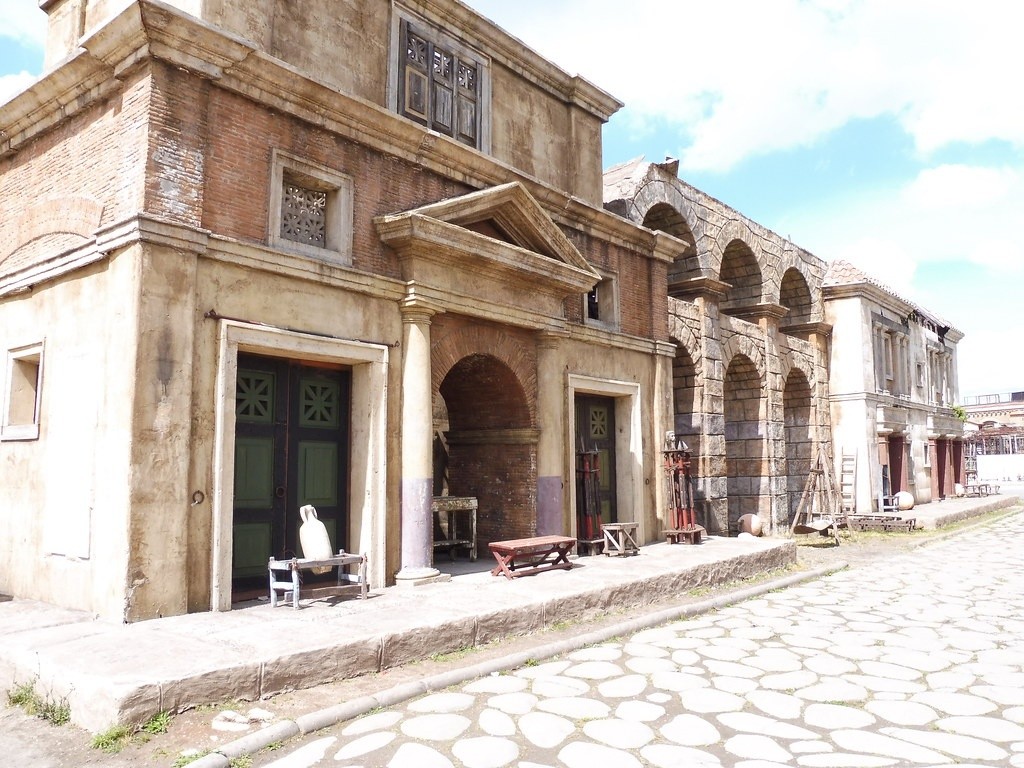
[488,535,576,581]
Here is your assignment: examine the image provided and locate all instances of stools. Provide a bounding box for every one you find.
[600,522,640,558]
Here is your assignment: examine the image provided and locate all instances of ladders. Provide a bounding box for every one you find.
[837,446,858,514]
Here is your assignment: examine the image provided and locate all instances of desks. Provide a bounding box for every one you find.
[433,496,479,564]
[965,484,989,498]
